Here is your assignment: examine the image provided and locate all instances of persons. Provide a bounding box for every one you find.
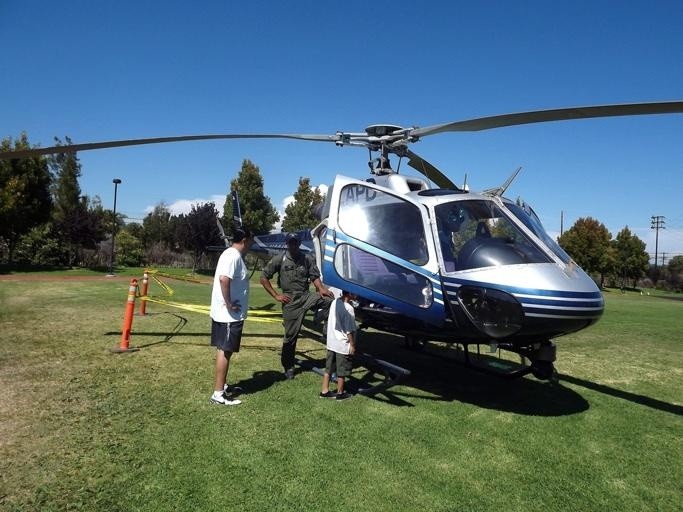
[319,290,357,402]
[260,232,335,379]
[210,227,251,406]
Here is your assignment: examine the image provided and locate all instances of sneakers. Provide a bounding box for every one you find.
[285,366,295,380]
[319,391,351,401]
[209,383,243,406]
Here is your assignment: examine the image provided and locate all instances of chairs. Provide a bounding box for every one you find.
[363,222,419,260]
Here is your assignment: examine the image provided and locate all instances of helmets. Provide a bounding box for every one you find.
[284,232,302,246]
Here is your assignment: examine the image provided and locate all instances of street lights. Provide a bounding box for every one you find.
[106,178,121,277]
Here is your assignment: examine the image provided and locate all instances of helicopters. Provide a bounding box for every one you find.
[0,97,683,383]
